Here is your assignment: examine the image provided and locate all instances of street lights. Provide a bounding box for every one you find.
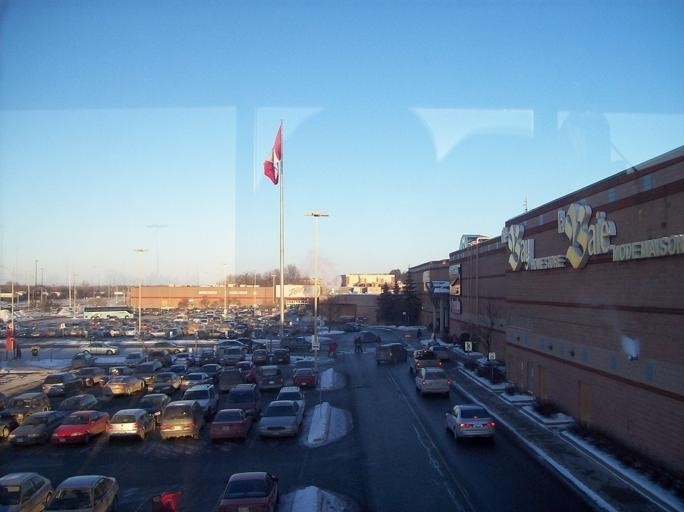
[134,245,150,334]
[6,255,127,304]
[5,262,17,359]
[223,261,233,311]
[250,268,258,306]
[304,210,329,371]
[269,273,277,304]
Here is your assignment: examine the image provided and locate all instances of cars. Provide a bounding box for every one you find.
[444,405,497,442]
[0,472,54,512]
[408,338,451,397]
[356,330,381,343]
[218,472,280,512]
[42,474,120,512]
[342,323,360,332]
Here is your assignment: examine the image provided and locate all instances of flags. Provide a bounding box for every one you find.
[263,124,283,185]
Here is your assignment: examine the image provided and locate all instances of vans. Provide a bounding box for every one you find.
[375,342,407,364]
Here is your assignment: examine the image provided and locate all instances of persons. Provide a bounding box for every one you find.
[327,342,338,358]
[153,495,172,512]
[246,362,256,383]
[16,343,22,360]
[353,336,362,353]
[416,328,423,346]
